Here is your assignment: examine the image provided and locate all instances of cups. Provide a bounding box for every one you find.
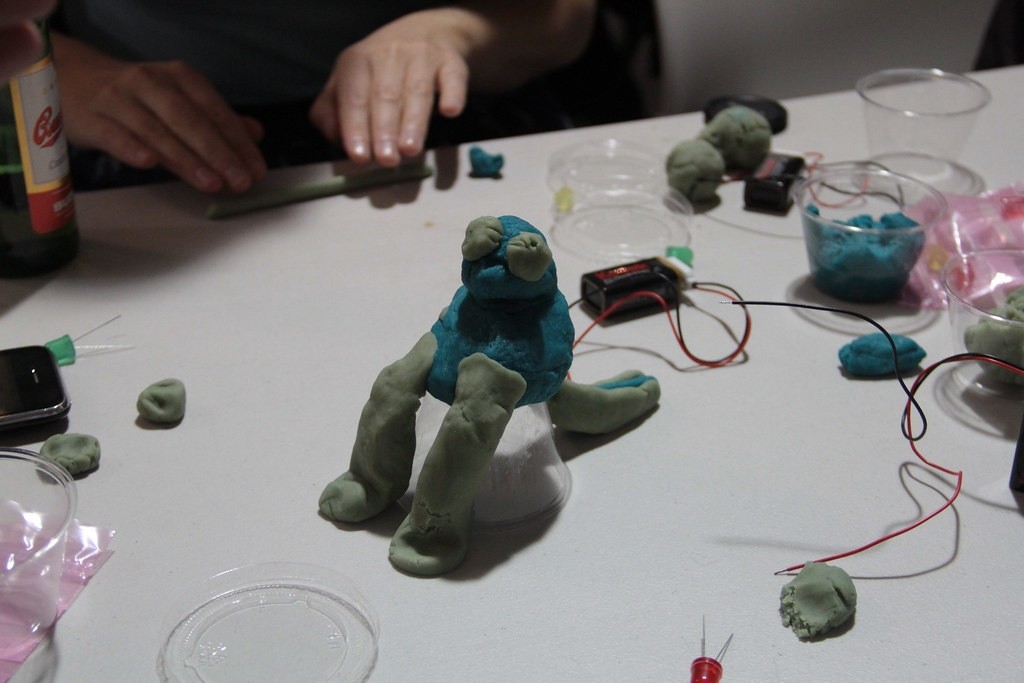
[470,402,572,528]
[937,247,1024,402]
[546,136,669,248]
[0,447,79,683]
[793,170,949,301]
[855,69,994,175]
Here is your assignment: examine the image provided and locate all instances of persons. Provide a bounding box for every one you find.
[35,0,599,197]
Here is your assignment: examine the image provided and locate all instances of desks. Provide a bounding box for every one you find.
[0,63,1024,681]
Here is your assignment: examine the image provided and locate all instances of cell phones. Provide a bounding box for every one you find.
[0,345,72,432]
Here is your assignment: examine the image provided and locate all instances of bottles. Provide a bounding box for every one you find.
[1,0,79,277]
